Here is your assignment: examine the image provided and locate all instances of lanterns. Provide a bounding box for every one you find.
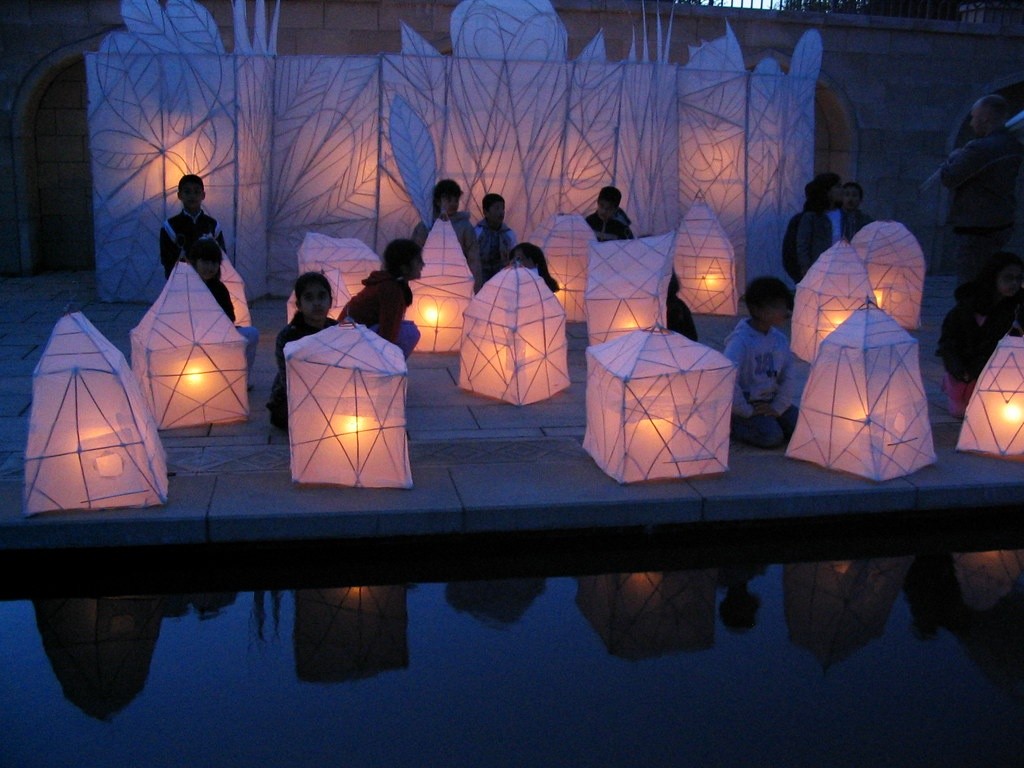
[23,249,254,517]
[583,326,735,482]
[540,198,738,346]
[285,232,571,409]
[791,239,874,364]
[955,333,1024,461]
[850,219,926,329]
[30,551,1024,727]
[285,317,414,489]
[784,303,938,483]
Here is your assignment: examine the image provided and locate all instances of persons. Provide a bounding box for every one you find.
[938,253,1024,418]
[721,277,799,446]
[161,175,227,278]
[940,96,1024,281]
[338,238,426,360]
[666,275,698,340]
[841,182,876,241]
[586,186,632,241]
[191,238,258,390]
[781,173,848,282]
[410,179,560,292]
[268,272,339,432]
